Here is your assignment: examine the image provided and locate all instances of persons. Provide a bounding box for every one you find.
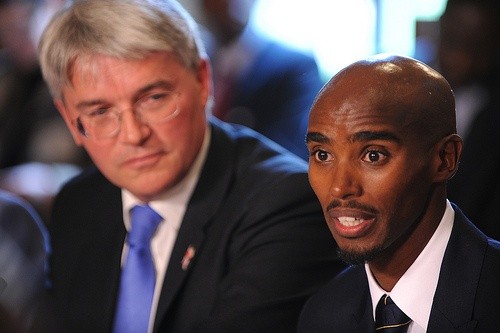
[298,52,500,333]
[30,0,336,332]
[437,0,500,241]
[175,0,325,161]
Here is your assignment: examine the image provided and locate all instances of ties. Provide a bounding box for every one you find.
[375,294,410,333]
[112,204,164,333]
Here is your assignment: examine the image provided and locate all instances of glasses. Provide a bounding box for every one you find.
[78,79,178,137]
